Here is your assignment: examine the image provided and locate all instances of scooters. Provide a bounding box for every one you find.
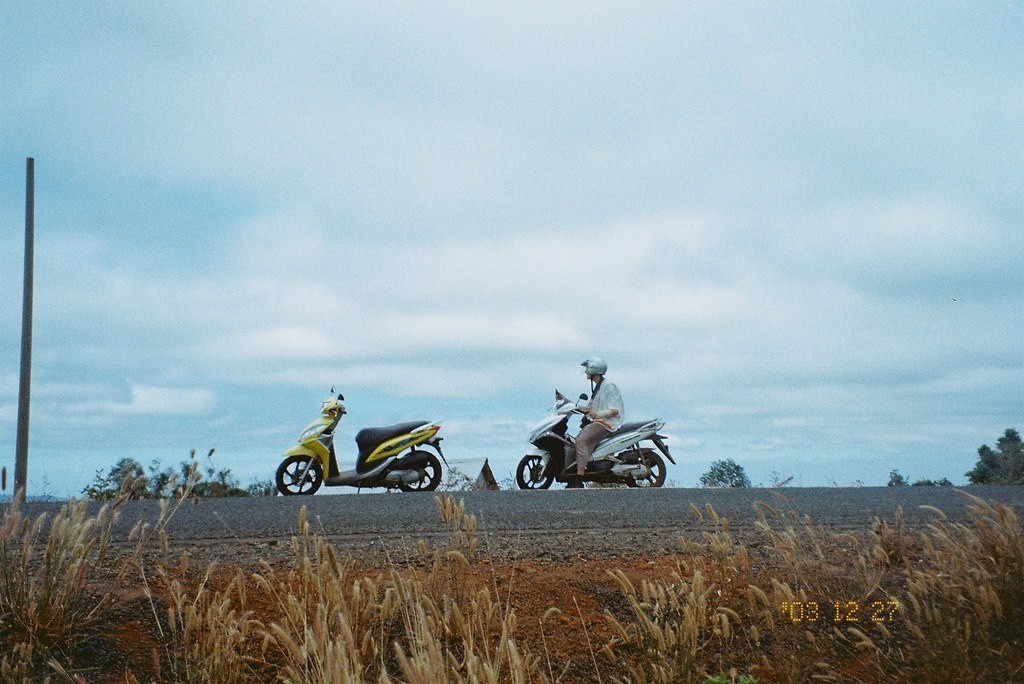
[275,385,449,496]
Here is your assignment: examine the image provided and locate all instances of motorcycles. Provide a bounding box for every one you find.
[516,390,676,490]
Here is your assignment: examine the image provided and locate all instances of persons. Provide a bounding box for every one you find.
[553,356,625,490]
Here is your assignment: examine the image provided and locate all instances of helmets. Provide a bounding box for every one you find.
[582,357,607,374]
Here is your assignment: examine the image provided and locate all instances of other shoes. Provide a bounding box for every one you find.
[566,482,584,488]
